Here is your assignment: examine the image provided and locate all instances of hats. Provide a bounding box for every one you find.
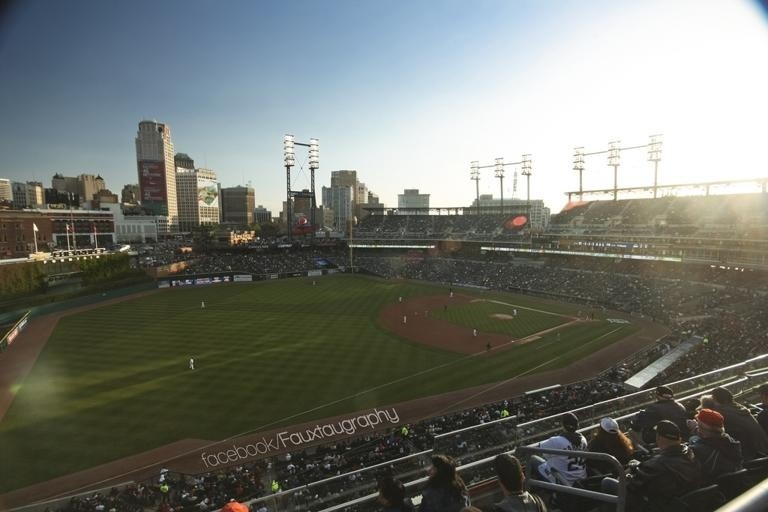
[698,408,724,427]
[655,386,674,398]
[653,420,682,441]
[601,416,620,434]
[563,411,579,429]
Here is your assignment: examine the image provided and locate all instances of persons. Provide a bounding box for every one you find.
[155,227,356,278]
[512,308,517,318]
[70,386,767,510]
[399,296,403,303]
[358,194,768,288]
[402,314,406,326]
[189,357,195,370]
[587,290,768,385]
[200,299,206,312]
[486,340,491,351]
[472,328,481,337]
[449,288,453,298]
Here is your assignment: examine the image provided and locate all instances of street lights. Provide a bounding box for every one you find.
[349,214,361,275]
[521,152,535,215]
[606,140,621,202]
[283,131,296,243]
[571,145,588,202]
[308,135,321,240]
[494,156,506,218]
[468,157,481,218]
[645,133,664,196]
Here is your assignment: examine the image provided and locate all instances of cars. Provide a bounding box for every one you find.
[112,239,195,268]
